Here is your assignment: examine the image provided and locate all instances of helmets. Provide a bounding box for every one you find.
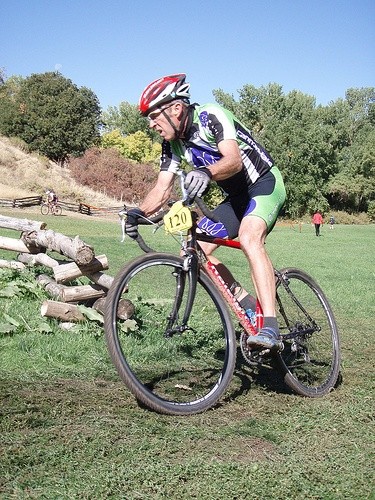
[138,73,190,116]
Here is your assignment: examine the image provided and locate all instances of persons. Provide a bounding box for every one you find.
[329,215,334,229]
[313,210,324,236]
[45,191,58,214]
[122,73,286,351]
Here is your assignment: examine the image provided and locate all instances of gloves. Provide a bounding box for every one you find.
[184,167,213,199]
[125,209,146,238]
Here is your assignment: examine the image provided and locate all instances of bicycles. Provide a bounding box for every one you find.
[103,167,342,415]
[41,202,62,216]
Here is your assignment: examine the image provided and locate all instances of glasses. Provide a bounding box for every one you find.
[149,103,177,120]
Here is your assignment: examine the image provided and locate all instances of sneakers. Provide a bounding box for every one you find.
[247,327,284,352]
